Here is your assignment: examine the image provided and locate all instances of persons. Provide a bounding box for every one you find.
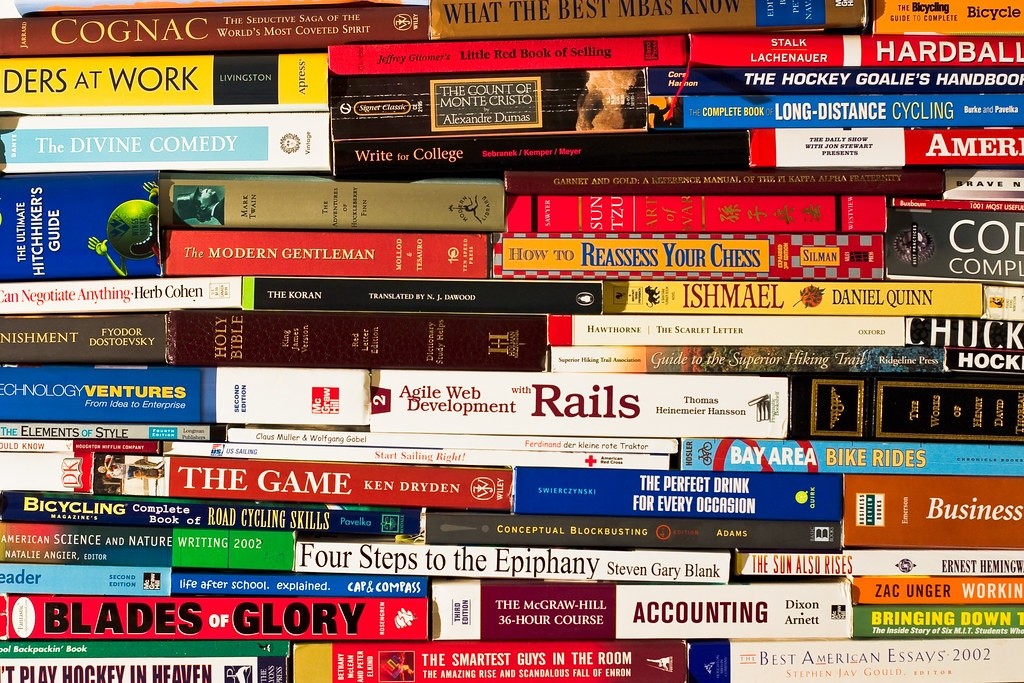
[177,186,224,225]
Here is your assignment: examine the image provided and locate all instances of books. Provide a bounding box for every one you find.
[0,0,1024,683]
